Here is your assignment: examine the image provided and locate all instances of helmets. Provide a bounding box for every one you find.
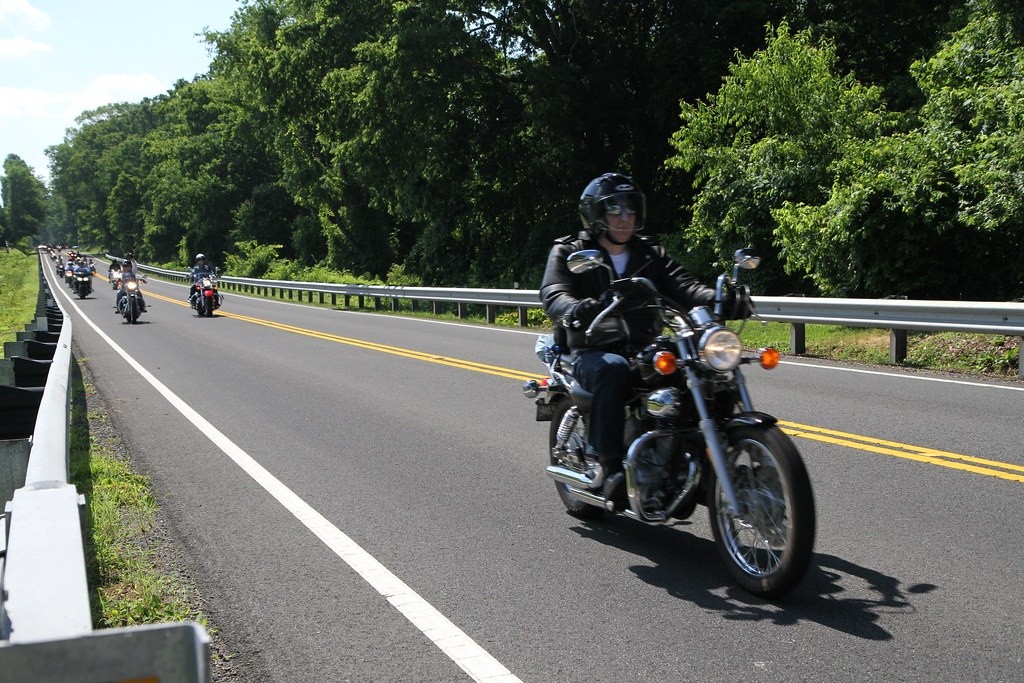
[578,174,645,234]
[196,253,205,260]
[57,253,132,275]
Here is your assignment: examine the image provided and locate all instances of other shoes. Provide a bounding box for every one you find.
[141,307,147,313]
[601,458,627,498]
[115,310,119,313]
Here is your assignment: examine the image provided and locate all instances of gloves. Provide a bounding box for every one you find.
[722,292,750,321]
[573,297,605,329]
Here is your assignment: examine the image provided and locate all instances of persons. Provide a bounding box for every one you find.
[46,243,97,294]
[189,254,220,307]
[108,258,147,312]
[540,175,752,512]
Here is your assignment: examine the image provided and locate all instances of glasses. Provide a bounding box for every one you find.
[601,204,637,215]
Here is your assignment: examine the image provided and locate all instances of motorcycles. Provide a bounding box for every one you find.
[520,246,817,603]
[45,246,96,299]
[112,274,150,324]
[186,266,225,317]
[108,269,122,290]
